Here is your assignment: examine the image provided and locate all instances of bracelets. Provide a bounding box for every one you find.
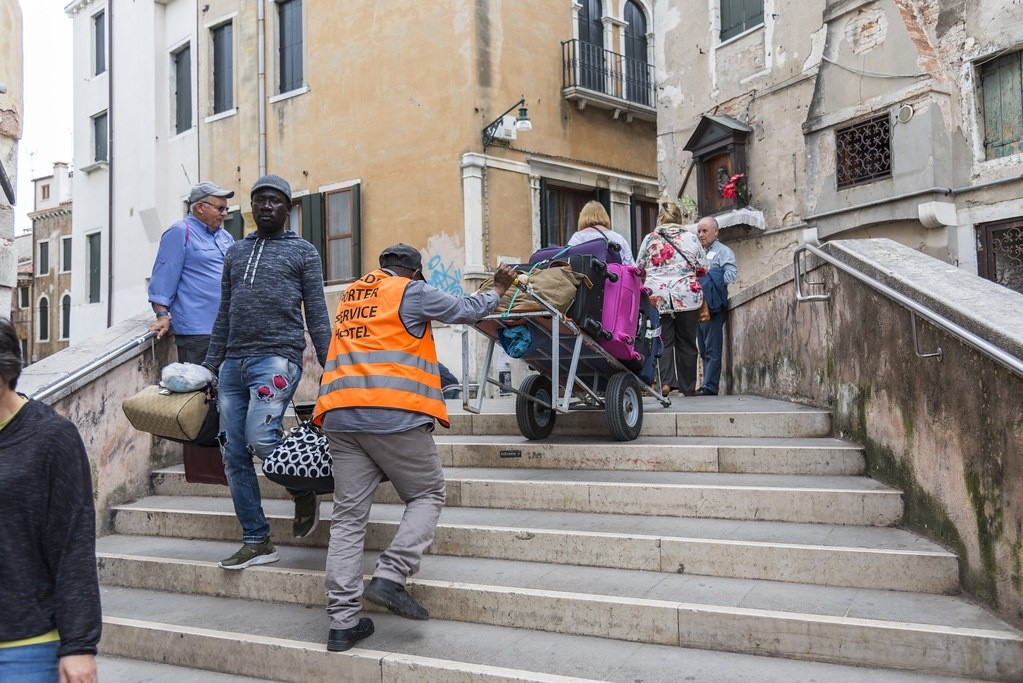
[155,310,167,318]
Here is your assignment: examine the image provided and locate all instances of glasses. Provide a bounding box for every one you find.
[202,201,229,213]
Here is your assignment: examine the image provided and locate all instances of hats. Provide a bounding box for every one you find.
[378,243,425,282]
[250,175,291,203]
[188,182,235,204]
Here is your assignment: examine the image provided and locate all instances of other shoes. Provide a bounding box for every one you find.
[661,384,670,395]
[698,387,715,395]
[682,390,700,397]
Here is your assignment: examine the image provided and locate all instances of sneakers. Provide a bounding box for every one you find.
[327,617,375,651]
[362,577,428,619]
[293,493,322,539]
[219,538,280,569]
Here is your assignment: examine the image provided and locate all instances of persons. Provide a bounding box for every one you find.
[565,199,637,265]
[199,174,338,568]
[691,216,737,395]
[147,179,238,366]
[634,201,708,396]
[320,243,517,652]
[0,315,102,682]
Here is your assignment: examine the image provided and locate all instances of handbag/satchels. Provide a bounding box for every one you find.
[122,362,224,448]
[697,296,709,322]
[262,411,335,492]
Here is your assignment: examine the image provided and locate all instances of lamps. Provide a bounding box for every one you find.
[482,94,532,151]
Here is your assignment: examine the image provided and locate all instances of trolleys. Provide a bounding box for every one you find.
[461,276,673,441]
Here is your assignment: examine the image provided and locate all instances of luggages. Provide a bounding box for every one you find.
[476,238,664,386]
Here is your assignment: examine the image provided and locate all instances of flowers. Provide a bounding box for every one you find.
[723,172,754,206]
